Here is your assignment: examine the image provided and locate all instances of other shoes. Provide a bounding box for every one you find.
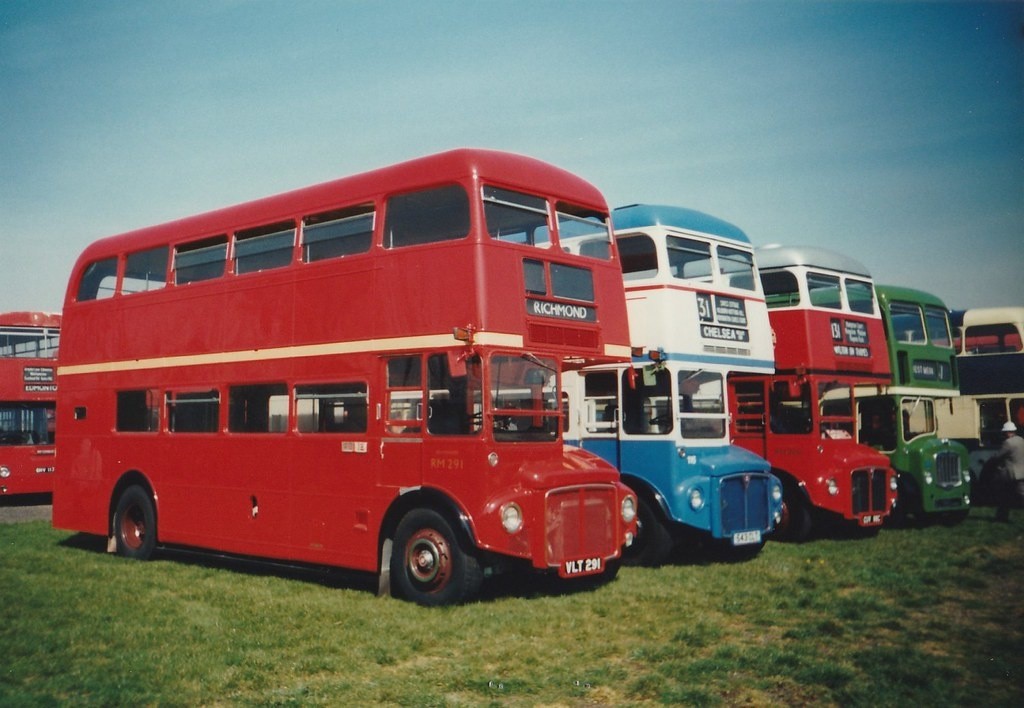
[992,518,1008,522]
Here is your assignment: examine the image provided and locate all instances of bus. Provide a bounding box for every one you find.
[0,310,63,500]
[625,239,1024,543]
[50,149,639,609]
[385,202,782,568]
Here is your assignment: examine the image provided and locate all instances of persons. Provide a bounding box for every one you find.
[990,421,1024,523]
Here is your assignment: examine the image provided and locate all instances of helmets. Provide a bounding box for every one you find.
[1002,421,1017,432]
